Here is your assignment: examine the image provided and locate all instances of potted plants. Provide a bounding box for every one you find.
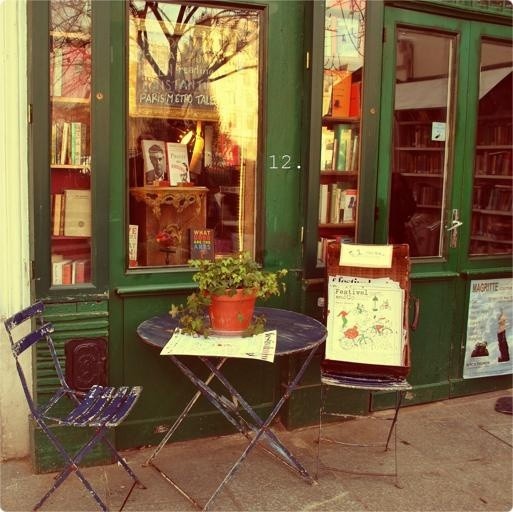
[170,250,288,340]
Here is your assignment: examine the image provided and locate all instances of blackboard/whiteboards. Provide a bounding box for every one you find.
[190,228,215,259]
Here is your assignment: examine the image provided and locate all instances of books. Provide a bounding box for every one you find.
[128,224,139,267]
[188,227,215,261]
[51,37,92,286]
[165,141,190,188]
[141,137,168,187]
[396,116,512,258]
[317,13,361,270]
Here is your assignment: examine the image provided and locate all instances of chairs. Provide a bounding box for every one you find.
[3,301,146,512]
[315,294,412,491]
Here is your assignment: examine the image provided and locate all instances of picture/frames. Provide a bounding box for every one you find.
[128,17,240,123]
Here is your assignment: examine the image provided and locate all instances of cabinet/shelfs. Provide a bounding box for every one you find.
[49,32,93,242]
[316,8,368,269]
[394,113,513,255]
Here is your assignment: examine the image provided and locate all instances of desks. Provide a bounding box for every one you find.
[136,307,328,512]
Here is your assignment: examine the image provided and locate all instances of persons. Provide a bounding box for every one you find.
[494,309,510,363]
[390,170,416,245]
[146,145,167,185]
